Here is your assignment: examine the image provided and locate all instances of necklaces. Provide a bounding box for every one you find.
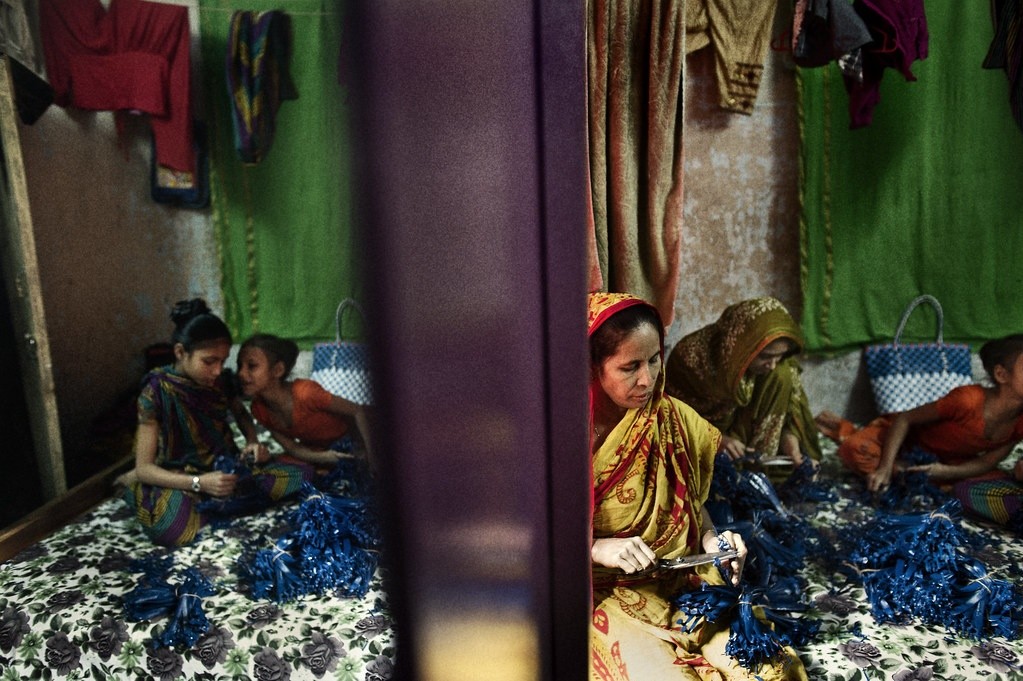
[593,422,605,445]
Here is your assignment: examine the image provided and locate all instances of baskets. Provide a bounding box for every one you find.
[309,299,376,406]
[864,293,976,415]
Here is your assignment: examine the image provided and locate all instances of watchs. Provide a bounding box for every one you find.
[191,477,201,494]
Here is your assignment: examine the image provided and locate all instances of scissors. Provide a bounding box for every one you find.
[743,447,794,466]
[613,550,740,588]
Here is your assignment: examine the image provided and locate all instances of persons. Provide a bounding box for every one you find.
[817,332,1023,491]
[114,297,305,548]
[589,290,809,681]
[235,333,390,495]
[666,297,824,494]
